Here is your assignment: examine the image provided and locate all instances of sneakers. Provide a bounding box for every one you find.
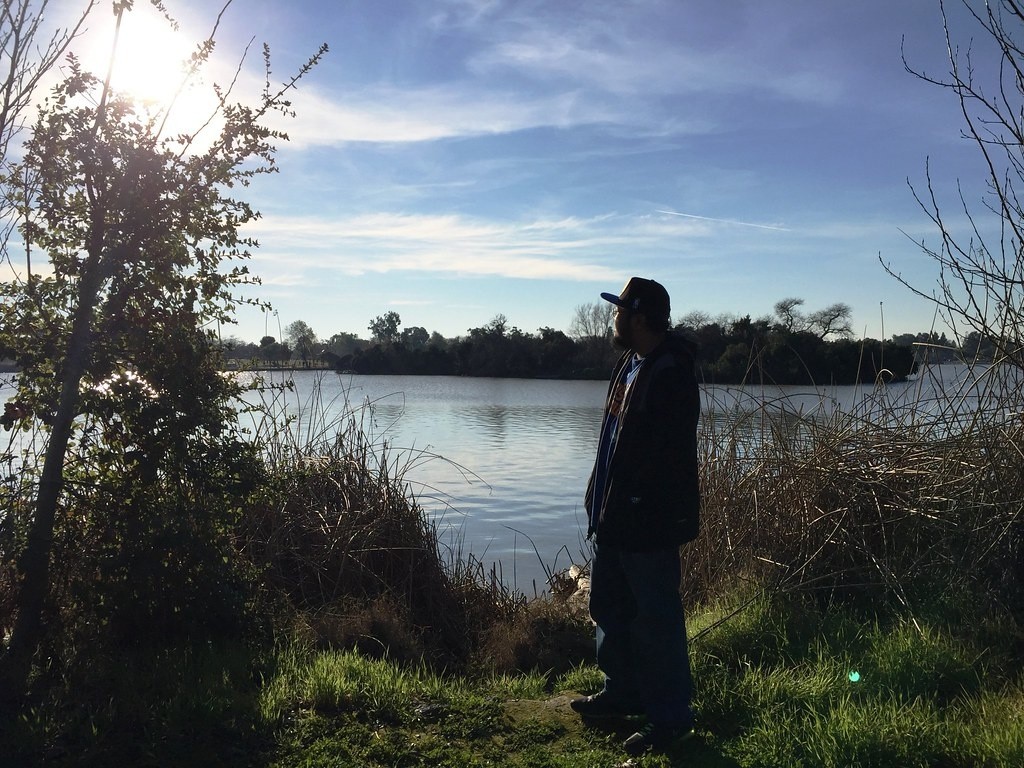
[623,715,696,758]
[571,679,648,721]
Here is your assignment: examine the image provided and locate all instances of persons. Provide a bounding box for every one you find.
[569,277,704,756]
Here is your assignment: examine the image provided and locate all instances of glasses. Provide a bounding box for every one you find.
[613,307,623,317]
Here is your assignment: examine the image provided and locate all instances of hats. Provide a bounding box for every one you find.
[601,277,670,314]
